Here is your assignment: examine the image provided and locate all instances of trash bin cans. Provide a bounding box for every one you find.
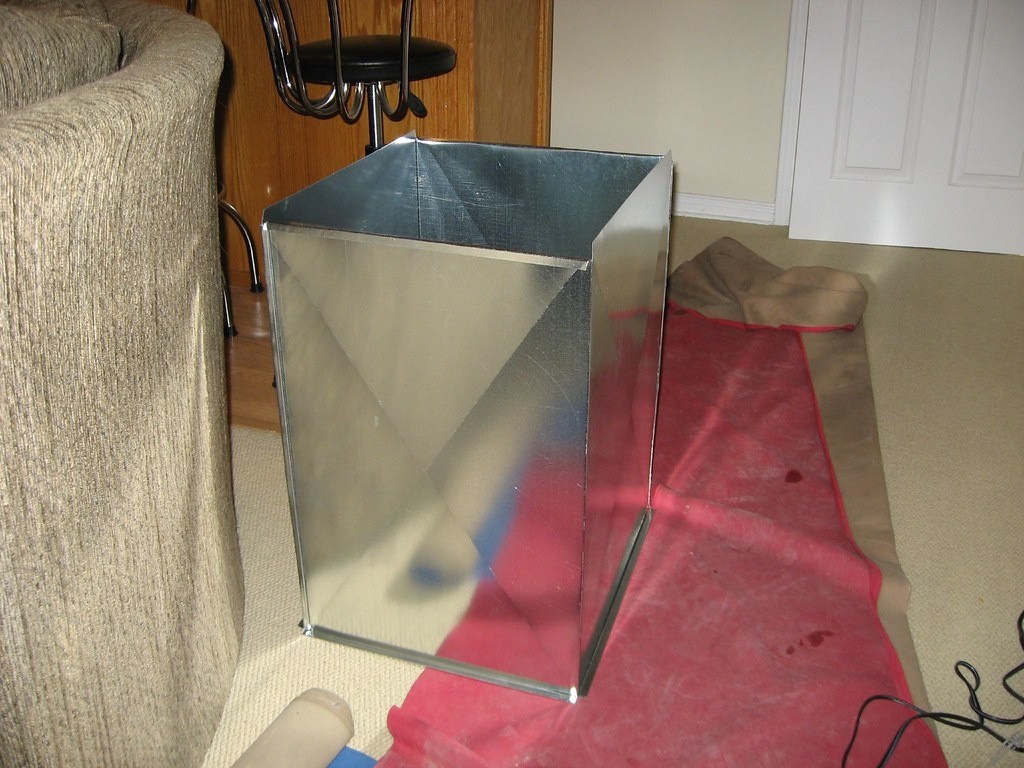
[258,129,676,705]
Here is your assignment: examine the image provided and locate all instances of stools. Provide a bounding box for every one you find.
[253,0,456,158]
[182,1,265,339]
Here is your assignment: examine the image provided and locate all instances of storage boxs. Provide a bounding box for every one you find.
[261,131,676,703]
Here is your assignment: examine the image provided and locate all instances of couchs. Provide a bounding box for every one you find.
[2,1,245,767]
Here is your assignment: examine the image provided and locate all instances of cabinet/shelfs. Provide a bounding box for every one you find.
[154,1,551,291]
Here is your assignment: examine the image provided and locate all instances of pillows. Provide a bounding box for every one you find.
[3,8,121,114]
